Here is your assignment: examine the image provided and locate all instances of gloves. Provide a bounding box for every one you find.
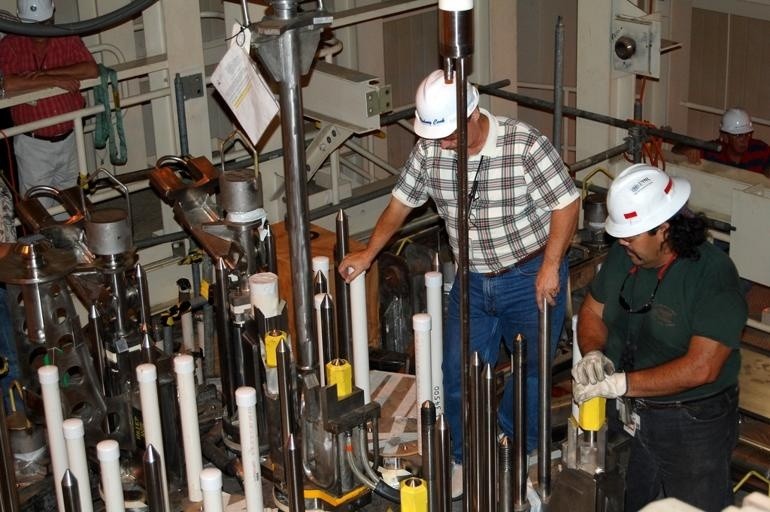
[571,351,627,405]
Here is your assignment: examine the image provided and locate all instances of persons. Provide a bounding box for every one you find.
[672,108,770,178]
[571,164,748,512]
[0,0,98,221]
[338,69,581,465]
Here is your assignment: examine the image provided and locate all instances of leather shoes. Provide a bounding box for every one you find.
[451,464,463,502]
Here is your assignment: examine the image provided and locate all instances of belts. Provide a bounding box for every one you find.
[23,129,73,143]
[484,245,546,279]
[633,379,739,411]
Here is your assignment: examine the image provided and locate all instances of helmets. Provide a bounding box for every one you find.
[413,69,480,139]
[16,0,55,24]
[603,163,690,239]
[718,108,754,135]
